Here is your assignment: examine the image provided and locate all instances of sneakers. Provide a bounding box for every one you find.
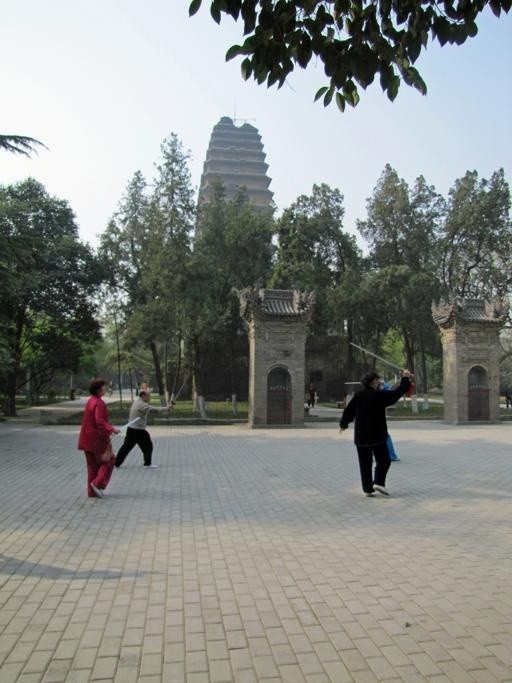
[144,463,158,469]
[90,482,103,496]
[361,484,391,495]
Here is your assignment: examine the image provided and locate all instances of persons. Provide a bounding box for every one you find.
[113,389,173,469]
[79,378,122,497]
[374,375,398,461]
[339,367,413,500]
[504,382,512,410]
[306,381,316,407]
